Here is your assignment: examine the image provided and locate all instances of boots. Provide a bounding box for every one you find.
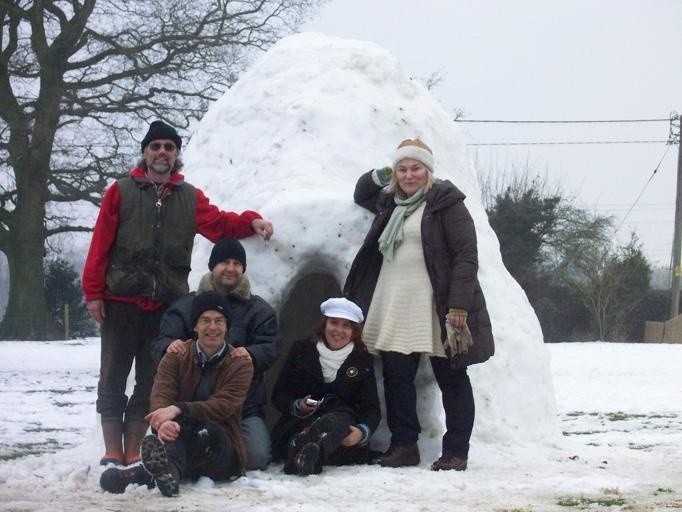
[100,417,123,463]
[123,413,149,466]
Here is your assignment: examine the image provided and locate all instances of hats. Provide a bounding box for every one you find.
[142,120,182,153]
[192,291,231,332]
[321,298,364,323]
[392,138,434,171]
[209,236,246,274]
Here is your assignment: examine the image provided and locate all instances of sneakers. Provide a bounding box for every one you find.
[140,435,181,498]
[297,443,319,476]
[294,413,337,451]
[99,465,154,495]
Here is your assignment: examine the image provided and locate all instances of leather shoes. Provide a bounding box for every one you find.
[432,454,467,470]
[372,442,420,467]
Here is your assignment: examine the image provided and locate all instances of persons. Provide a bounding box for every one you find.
[152,237,283,470]
[82,120,273,466]
[270,297,384,477]
[343,138,494,471]
[100,292,254,498]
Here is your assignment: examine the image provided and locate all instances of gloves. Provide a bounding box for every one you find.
[445,314,475,369]
[372,166,394,186]
